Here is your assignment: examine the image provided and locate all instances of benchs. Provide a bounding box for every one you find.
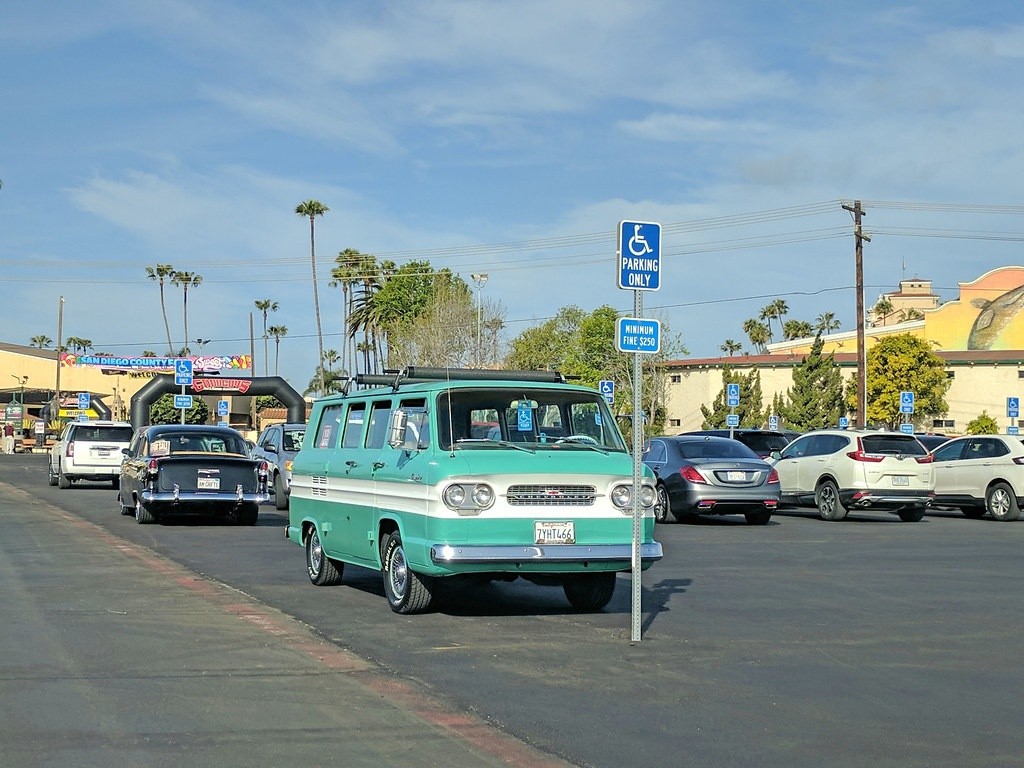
[21,439,57,453]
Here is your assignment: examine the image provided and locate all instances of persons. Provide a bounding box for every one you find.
[42,395,66,420]
[3,421,16,455]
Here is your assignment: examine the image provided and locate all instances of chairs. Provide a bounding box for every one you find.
[974,444,993,457]
[284,434,294,448]
[541,426,568,442]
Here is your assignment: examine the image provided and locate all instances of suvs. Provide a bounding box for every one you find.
[676,427,814,458]
[249,421,307,510]
[761,424,938,523]
[913,431,964,457]
[471,419,563,443]
[926,431,1024,522]
[47,418,135,489]
[117,423,270,527]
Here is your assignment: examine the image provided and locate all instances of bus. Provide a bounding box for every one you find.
[282,365,666,615]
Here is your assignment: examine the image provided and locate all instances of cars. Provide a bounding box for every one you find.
[629,432,781,525]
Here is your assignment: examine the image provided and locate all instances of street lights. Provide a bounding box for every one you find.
[53,294,68,430]
[191,338,211,410]
[470,273,490,369]
[11,374,29,435]
